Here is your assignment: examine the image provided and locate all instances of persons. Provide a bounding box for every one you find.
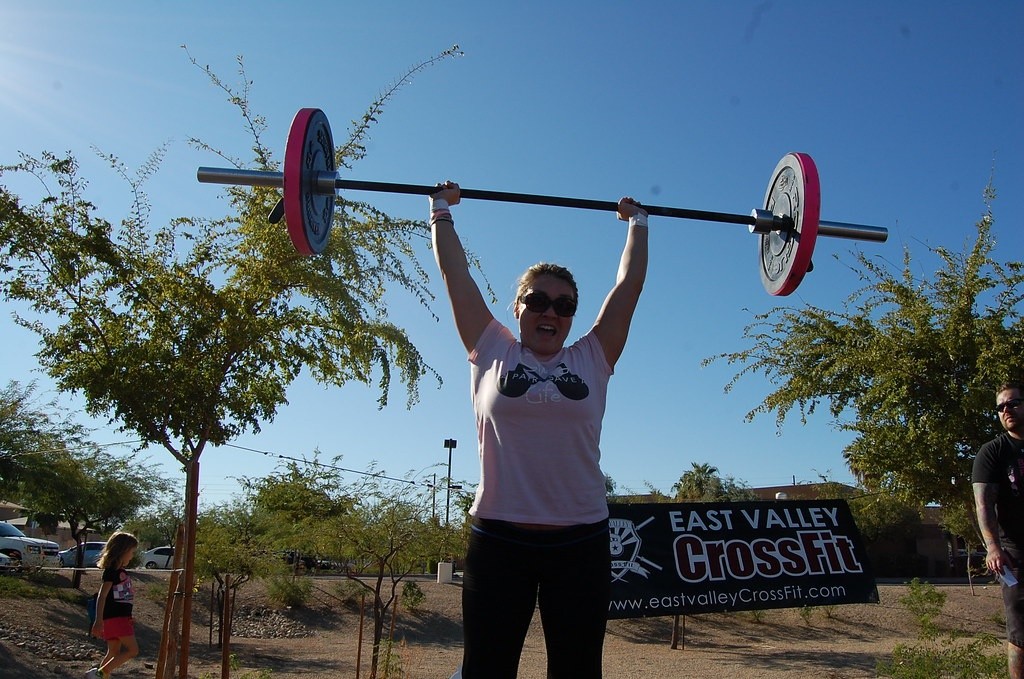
[428,179,650,679]
[971,379,1024,679]
[83,532,139,679]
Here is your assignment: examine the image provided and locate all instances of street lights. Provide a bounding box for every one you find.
[443,439,462,563]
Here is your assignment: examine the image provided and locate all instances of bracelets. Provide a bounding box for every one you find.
[628,213,648,228]
[430,198,451,212]
[429,210,455,226]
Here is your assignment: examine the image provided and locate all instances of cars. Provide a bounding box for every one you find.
[58,542,108,568]
[0,521,60,568]
[139,547,184,569]
[0,553,23,576]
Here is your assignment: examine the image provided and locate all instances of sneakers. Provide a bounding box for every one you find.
[85,667,104,679]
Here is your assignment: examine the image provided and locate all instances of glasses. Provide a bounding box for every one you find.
[519,292,577,317]
[995,398,1024,411]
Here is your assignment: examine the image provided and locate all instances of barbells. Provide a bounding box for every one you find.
[196,103,893,299]
